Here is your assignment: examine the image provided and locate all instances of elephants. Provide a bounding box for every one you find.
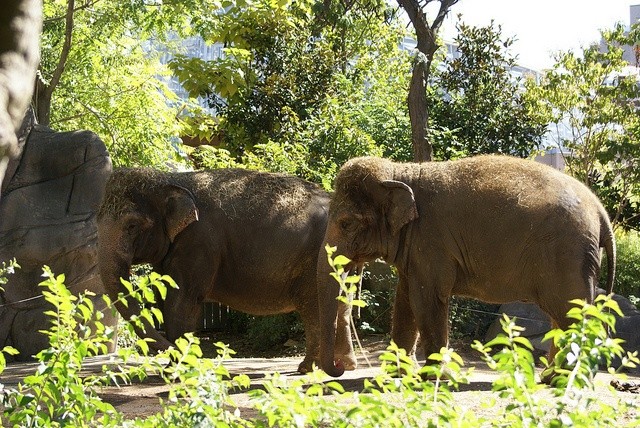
[0,0,42,193]
[316,154,616,386]
[95,168,365,378]
